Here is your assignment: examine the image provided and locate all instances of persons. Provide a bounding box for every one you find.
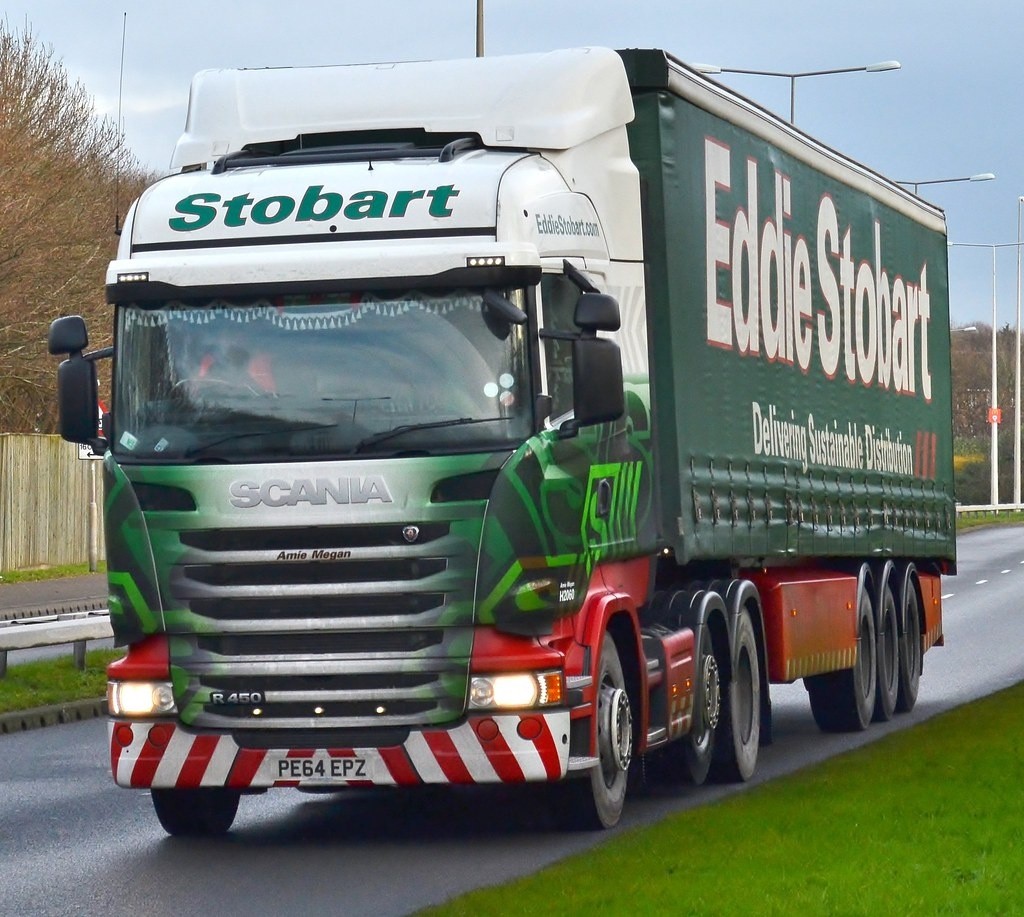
[177,330,281,398]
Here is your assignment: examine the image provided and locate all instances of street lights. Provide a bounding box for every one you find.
[896,174,996,196]
[948,242,1024,514]
[691,60,902,126]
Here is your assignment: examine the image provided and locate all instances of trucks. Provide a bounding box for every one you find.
[48,49,956,838]
